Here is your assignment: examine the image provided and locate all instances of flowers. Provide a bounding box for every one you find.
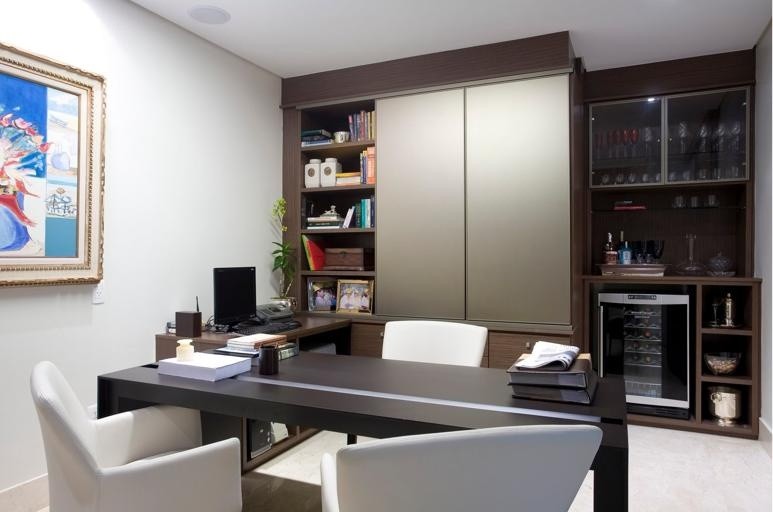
[271,198,294,296]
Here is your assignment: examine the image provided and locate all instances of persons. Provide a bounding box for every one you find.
[340,289,368,310]
[313,282,337,309]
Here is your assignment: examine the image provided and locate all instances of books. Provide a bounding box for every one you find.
[226,333,288,352]
[349,111,374,142]
[506,352,593,387]
[302,235,322,270]
[506,370,600,406]
[341,196,375,229]
[359,147,376,184]
[336,172,361,185]
[158,352,252,383]
[306,216,343,230]
[307,239,326,272]
[302,128,333,147]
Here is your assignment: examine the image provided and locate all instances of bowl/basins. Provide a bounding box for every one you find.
[705,351,741,374]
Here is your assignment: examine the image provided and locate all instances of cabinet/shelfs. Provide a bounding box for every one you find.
[299,98,377,276]
[373,74,575,326]
[580,83,757,437]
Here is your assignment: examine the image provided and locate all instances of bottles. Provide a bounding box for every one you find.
[604,231,632,265]
[321,158,342,187]
[626,306,661,362]
[305,159,320,188]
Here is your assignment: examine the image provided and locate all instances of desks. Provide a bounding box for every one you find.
[99,351,628,509]
[155,312,352,472]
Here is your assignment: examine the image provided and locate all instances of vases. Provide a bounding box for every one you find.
[274,299,298,315]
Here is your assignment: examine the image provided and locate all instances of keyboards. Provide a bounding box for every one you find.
[238,319,302,335]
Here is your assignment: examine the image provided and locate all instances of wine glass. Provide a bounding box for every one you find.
[594,127,660,159]
[669,120,745,152]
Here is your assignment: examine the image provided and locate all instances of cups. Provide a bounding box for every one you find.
[333,131,349,143]
[599,172,660,186]
[672,195,720,209]
[258,346,279,375]
[668,166,742,181]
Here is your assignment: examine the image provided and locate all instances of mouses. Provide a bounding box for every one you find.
[288,321,301,326]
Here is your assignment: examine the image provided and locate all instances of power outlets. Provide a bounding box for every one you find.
[93,285,105,303]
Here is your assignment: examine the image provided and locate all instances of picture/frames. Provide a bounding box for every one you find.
[0,43,107,288]
[338,281,372,315]
[309,280,334,310]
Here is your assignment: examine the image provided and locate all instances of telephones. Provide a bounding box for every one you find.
[252,303,293,323]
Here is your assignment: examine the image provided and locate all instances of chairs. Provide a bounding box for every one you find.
[31,359,243,512]
[320,425,602,509]
[347,319,488,444]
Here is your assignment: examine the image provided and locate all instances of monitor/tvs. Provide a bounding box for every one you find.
[211,265,258,334]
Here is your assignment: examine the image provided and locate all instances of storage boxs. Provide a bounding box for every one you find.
[325,247,364,267]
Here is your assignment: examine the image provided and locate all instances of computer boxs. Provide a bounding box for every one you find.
[249,419,273,458]
